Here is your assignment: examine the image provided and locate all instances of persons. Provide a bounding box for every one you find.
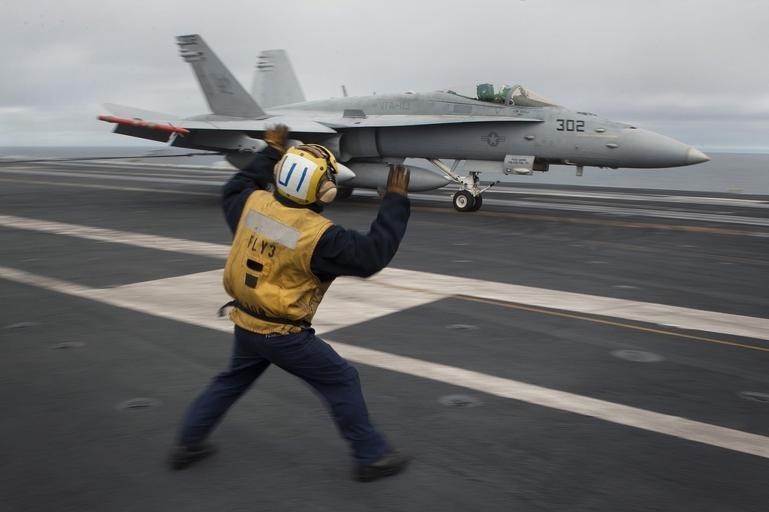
[159,120,423,482]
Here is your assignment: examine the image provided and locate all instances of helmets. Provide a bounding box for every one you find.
[272,143,338,208]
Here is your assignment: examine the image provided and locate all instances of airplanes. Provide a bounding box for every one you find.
[95,31,713,215]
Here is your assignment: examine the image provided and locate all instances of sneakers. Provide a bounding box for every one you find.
[164,439,218,472]
[352,449,409,483]
